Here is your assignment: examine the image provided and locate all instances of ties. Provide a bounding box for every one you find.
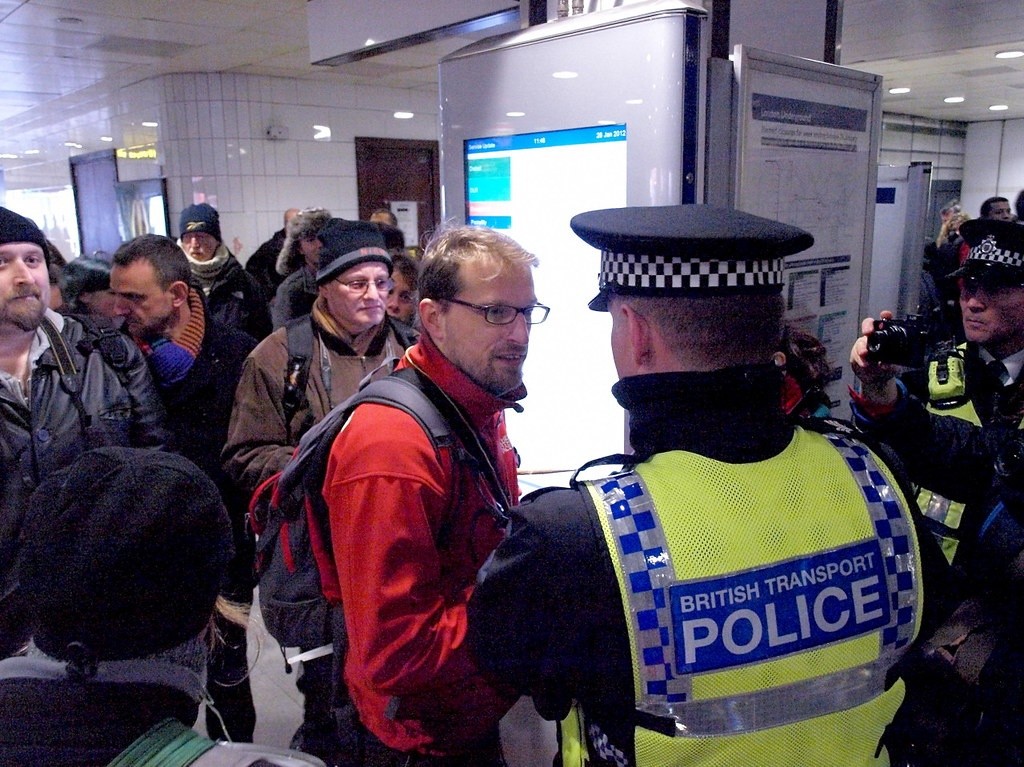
[986,359,1007,389]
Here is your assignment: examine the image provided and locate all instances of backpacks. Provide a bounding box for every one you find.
[247,354,508,646]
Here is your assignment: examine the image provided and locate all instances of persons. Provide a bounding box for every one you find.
[0,193,1024,766]
[319,231,552,766]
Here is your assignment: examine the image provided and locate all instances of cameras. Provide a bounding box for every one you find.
[867,312,928,369]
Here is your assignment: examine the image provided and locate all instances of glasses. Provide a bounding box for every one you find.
[334,278,397,291]
[419,295,550,325]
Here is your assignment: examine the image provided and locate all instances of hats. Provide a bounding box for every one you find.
[942,219,1024,288]
[180,203,221,243]
[570,203,816,312]
[276,207,333,276]
[17,447,229,661]
[0,205,50,269]
[317,218,394,286]
